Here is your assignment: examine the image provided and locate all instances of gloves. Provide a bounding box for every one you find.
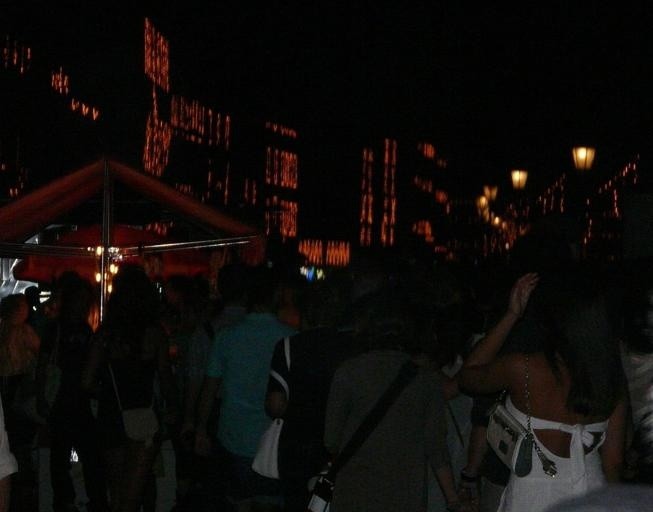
[458,468,480,511]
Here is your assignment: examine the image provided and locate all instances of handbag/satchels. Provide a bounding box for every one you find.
[485,403,533,478]
[251,417,284,481]
[306,462,335,512]
[121,407,160,442]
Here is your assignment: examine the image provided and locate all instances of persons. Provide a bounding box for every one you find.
[0,228,652,512]
